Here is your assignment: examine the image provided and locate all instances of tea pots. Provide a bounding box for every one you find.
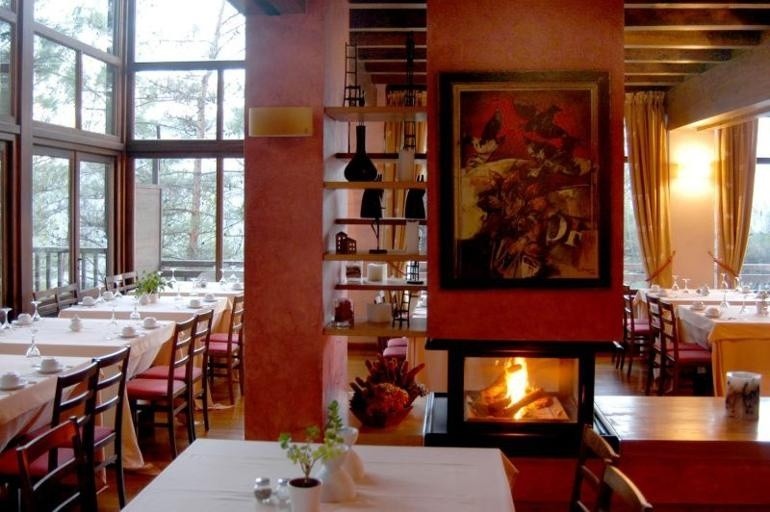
[69,314,84,332]
[138,292,152,306]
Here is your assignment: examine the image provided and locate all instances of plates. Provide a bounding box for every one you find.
[141,325,163,330]
[37,367,64,372]
[229,287,245,291]
[649,290,660,293]
[77,301,97,306]
[655,294,669,297]
[187,305,204,309]
[202,299,218,302]
[102,298,116,302]
[704,314,721,318]
[0,381,27,390]
[12,320,33,325]
[119,332,142,338]
[689,307,706,311]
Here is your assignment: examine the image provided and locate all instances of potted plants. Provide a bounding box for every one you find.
[277,397,351,512]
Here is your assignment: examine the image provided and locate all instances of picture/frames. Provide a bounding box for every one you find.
[433,67,614,293]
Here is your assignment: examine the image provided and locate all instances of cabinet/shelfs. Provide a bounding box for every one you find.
[323,107,432,339]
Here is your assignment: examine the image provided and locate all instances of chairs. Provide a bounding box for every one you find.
[594,464,653,511]
[644,291,706,384]
[199,330,244,345]
[611,316,647,371]
[126,314,197,460]
[134,307,215,440]
[26,343,134,510]
[0,359,102,492]
[126,269,141,296]
[55,283,82,311]
[199,293,246,406]
[32,287,60,317]
[657,298,713,396]
[103,273,126,296]
[0,414,95,512]
[620,283,662,379]
[570,421,625,510]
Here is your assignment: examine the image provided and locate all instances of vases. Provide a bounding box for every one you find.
[342,121,378,182]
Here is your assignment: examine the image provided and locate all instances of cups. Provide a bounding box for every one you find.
[144,319,156,325]
[168,267,177,288]
[651,284,660,290]
[25,330,41,360]
[692,301,704,307]
[233,283,242,289]
[132,302,142,325]
[113,280,122,303]
[205,293,215,299]
[682,279,691,299]
[1,309,11,334]
[32,300,43,322]
[40,359,58,369]
[0,373,22,384]
[672,274,679,297]
[720,273,729,292]
[174,286,184,305]
[190,298,201,306]
[219,268,228,290]
[122,326,136,334]
[658,289,667,294]
[95,285,106,309]
[229,265,240,284]
[735,277,743,293]
[18,313,32,321]
[111,307,117,334]
[706,308,719,314]
[104,292,113,299]
[719,293,732,314]
[739,296,749,314]
[83,296,93,303]
